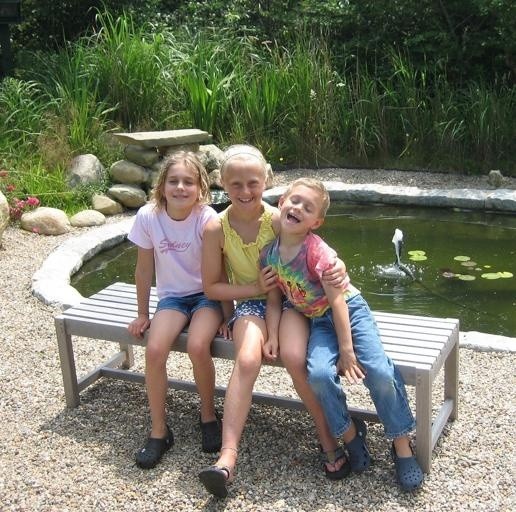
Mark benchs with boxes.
[54,282,459,473]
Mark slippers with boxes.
[198,465,230,498]
[199,411,222,453]
[320,417,369,480]
[391,440,424,491]
[135,424,175,469]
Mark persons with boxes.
[128,150,234,469]
[198,146,351,496]
[255,178,422,492]
